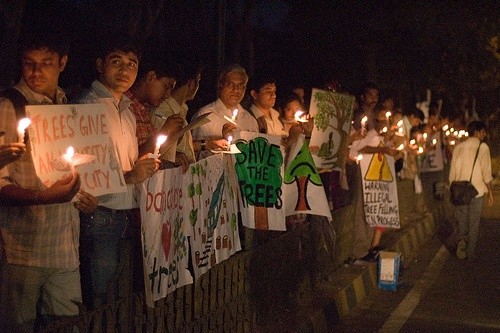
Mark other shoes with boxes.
[455,240,467,261]
[369,245,386,261]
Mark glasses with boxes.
[222,82,245,89]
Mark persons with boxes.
[0,24,99,333]
[449,121,494,259]
[136,56,491,328]
[74,33,162,333]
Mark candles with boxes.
[226,135,232,151]
[231,109,238,123]
[64,146,77,178]
[360,116,368,127]
[378,111,469,156]
[356,154,363,162]
[16,117,32,144]
[153,134,169,157]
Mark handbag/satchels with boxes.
[449,180,479,206]
